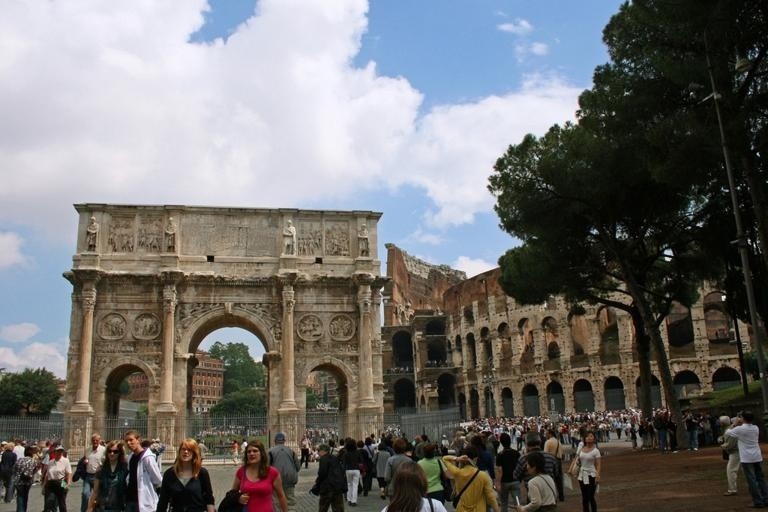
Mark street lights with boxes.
[682,16,766,408]
[483,373,495,419]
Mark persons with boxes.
[165,217,178,251]
[110,218,161,252]
[180,304,194,327]
[298,225,350,256]
[250,304,271,320]
[357,224,370,258]
[282,220,296,255]
[84,216,99,250]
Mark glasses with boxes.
[107,448,120,454]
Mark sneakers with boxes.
[348,500,356,506]
[748,502,765,508]
[358,487,369,497]
[625,437,698,454]
[234,462,240,466]
[561,437,621,448]
[723,490,737,496]
[380,492,386,499]
[300,465,308,469]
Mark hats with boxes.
[54,444,65,453]
[275,431,286,443]
[316,444,330,451]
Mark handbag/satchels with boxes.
[310,482,321,496]
[722,446,730,460]
[96,485,123,510]
[360,463,366,474]
[441,473,460,509]
[568,459,580,478]
